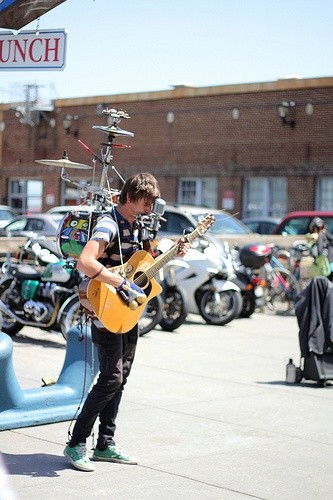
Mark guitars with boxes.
[78,213,216,335]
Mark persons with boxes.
[63,172,191,473]
[283,216,333,315]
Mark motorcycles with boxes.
[0,237,272,337]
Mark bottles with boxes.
[286,359,295,384]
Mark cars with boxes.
[0,205,333,297]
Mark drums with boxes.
[80,186,121,205]
[56,210,90,262]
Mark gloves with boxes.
[117,280,146,308]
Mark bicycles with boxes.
[224,242,304,315]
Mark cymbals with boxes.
[92,125,134,137]
[34,159,93,170]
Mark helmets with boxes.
[310,217,324,233]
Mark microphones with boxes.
[152,198,166,237]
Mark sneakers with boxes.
[93,444,138,465]
[63,441,95,471]
